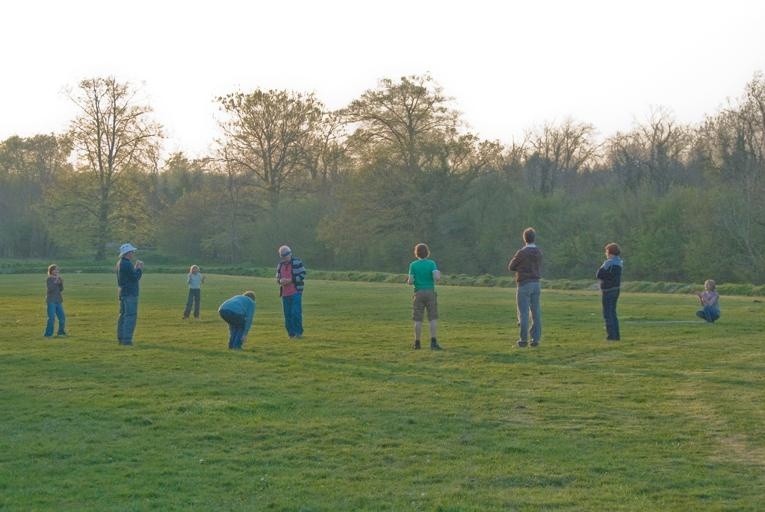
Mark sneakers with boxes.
[411,343,419,349]
[432,343,439,348]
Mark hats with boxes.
[119,244,136,256]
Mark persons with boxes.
[44,264,66,338]
[695,279,720,323]
[183,265,205,321]
[509,227,544,347]
[276,245,306,340]
[596,243,624,341]
[408,243,442,351]
[218,291,256,350]
[115,244,144,347]
